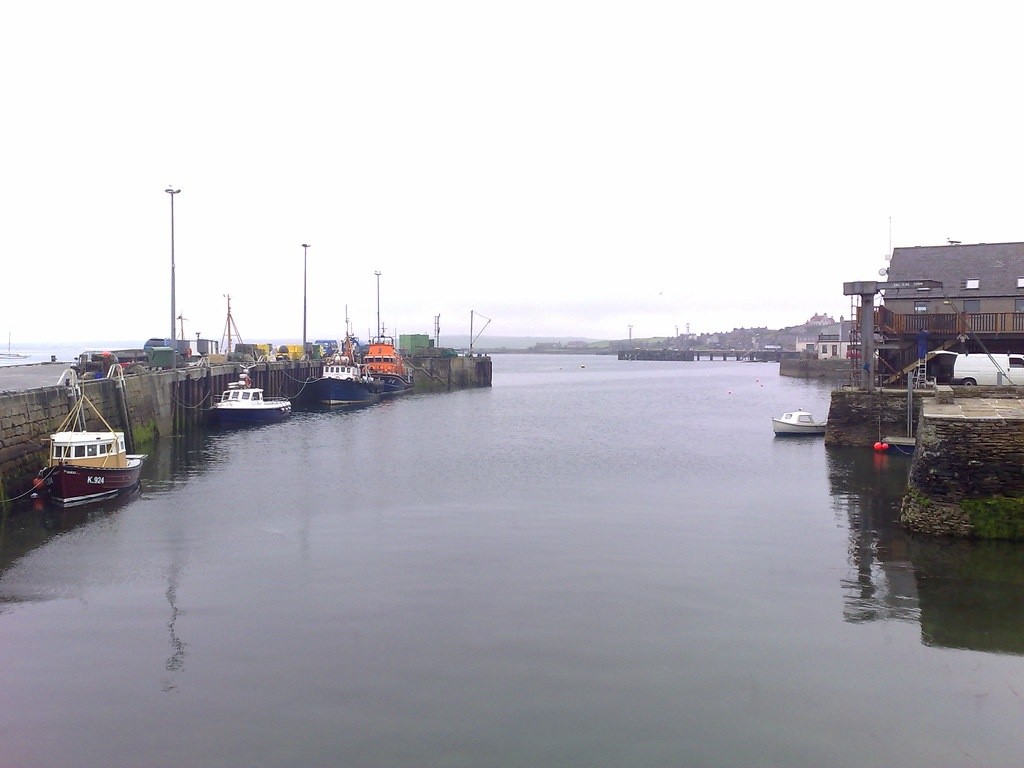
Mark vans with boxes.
[927,350,1024,385]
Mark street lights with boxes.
[374,270,382,342]
[164,188,182,371]
[301,243,311,356]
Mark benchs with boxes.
[934,385,954,404]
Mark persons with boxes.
[914,328,928,359]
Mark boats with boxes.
[310,329,385,406]
[209,364,292,422]
[362,334,414,392]
[771,410,827,435]
[31,393,148,513]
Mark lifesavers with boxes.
[244,377,252,389]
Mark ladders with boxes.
[917,354,927,388]
